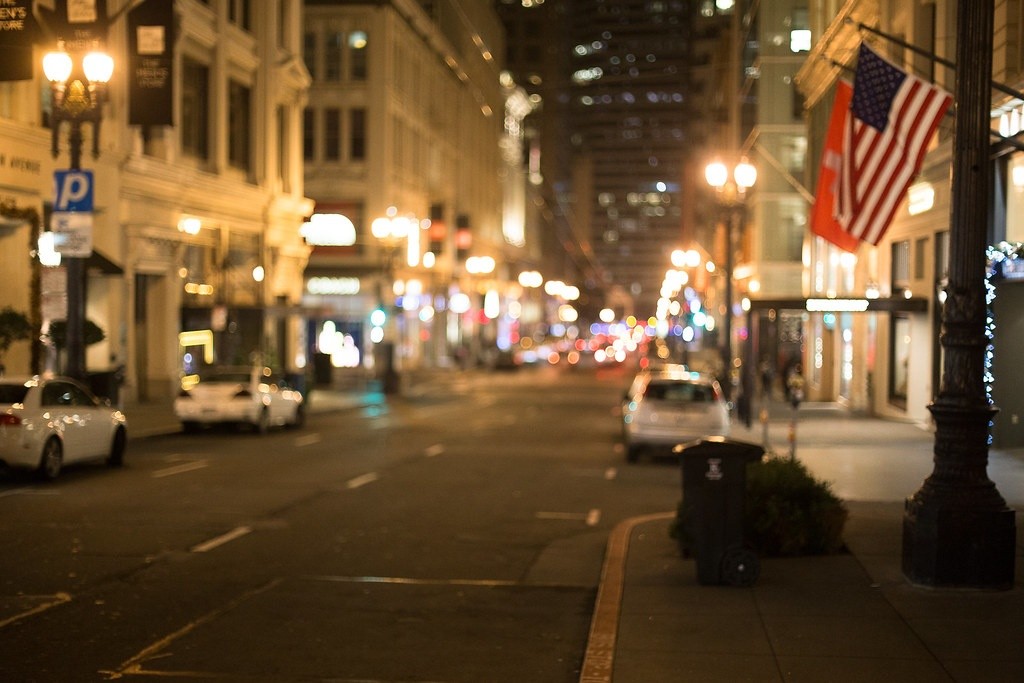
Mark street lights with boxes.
[43,51,114,382]
[705,159,757,405]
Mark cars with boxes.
[0,374,129,484]
[174,365,306,438]
[620,369,731,466]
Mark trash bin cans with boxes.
[89,372,120,407]
[674,436,766,586]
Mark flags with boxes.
[809,76,861,254]
[832,43,955,247]
